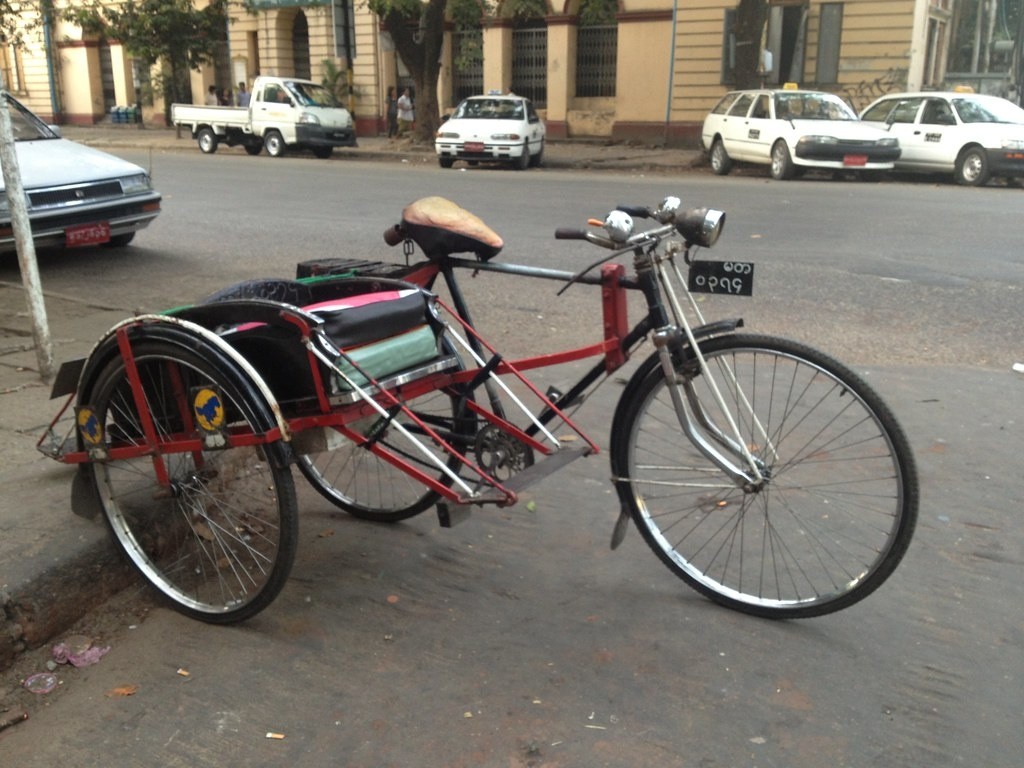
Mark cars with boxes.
[0,90,161,275]
[703,83,902,181]
[435,93,546,169]
[858,86,1024,186]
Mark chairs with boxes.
[776,100,796,119]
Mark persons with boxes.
[202,80,251,108]
[383,85,414,140]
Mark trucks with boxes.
[171,75,357,160]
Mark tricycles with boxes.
[39,196,920,625]
